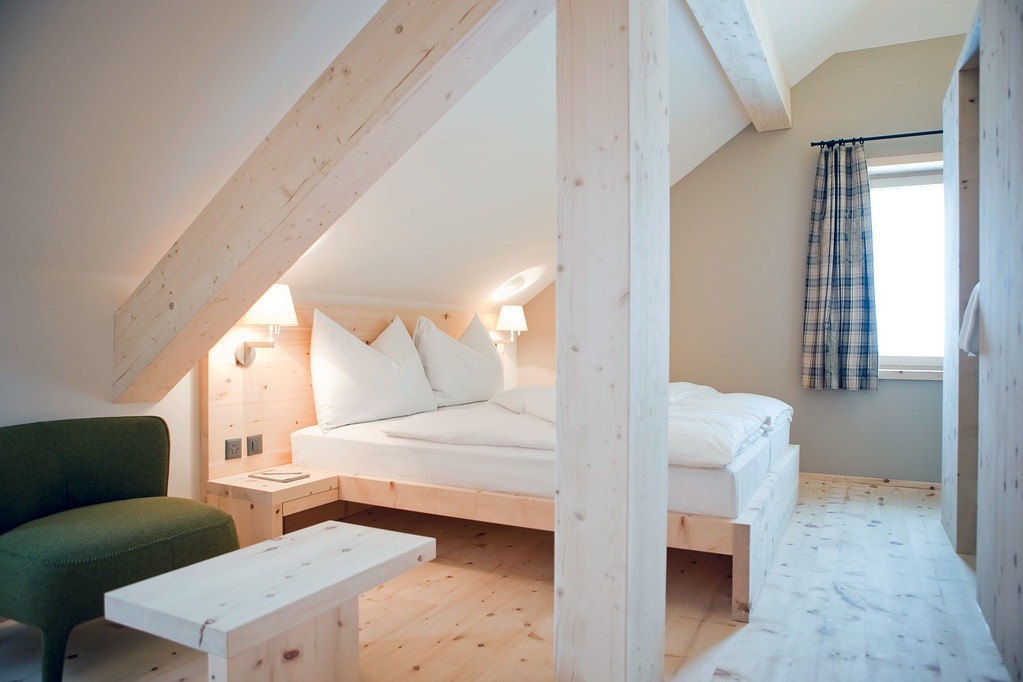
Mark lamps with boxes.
[234,283,297,368]
[493,306,529,347]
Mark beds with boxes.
[194,317,802,622]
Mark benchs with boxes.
[0,417,240,682]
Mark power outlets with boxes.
[225,438,242,460]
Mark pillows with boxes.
[414,315,503,407]
[309,309,437,436]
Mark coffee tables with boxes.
[103,520,435,682]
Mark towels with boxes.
[957,280,981,357]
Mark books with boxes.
[248,468,310,483]
